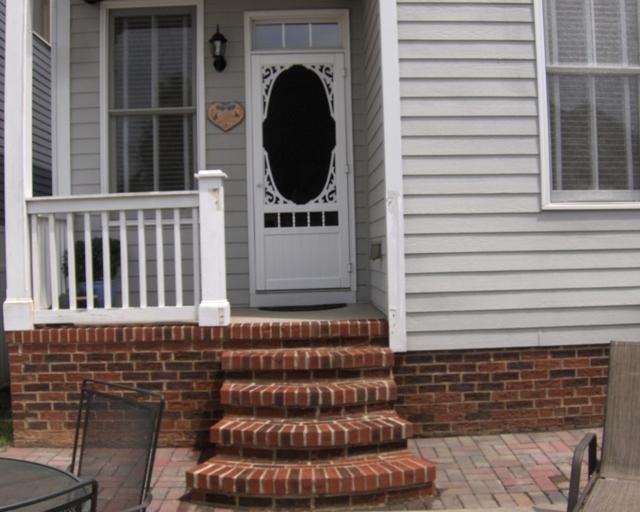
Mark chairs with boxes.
[2,479,98,512]
[566,338,638,511]
[66,378,167,511]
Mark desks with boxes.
[1,454,92,512]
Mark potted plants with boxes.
[62,235,121,307]
